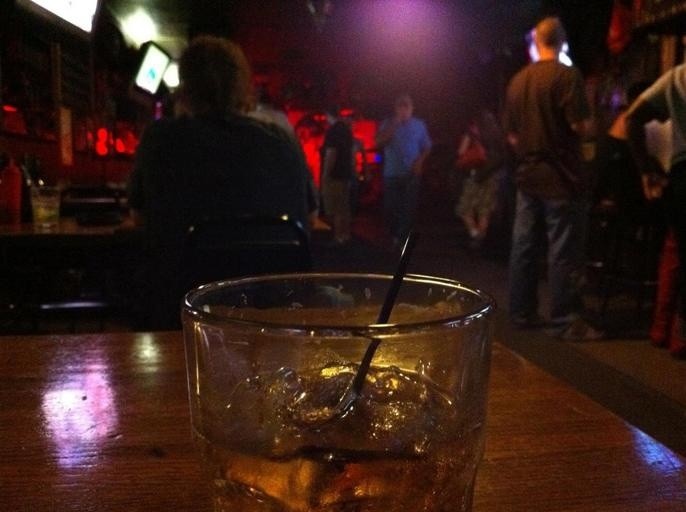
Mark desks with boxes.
[0,211,142,256]
[0,329,686,510]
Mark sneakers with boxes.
[511,311,543,328]
[323,237,354,251]
[555,319,606,342]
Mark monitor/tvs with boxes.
[524,27,574,67]
[15,0,104,42]
[133,41,171,95]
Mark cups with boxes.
[174,272,502,506]
[26,185,61,230]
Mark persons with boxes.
[373,94,436,265]
[128,37,320,332]
[317,104,357,253]
[341,117,370,227]
[451,18,685,366]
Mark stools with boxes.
[1,294,118,333]
